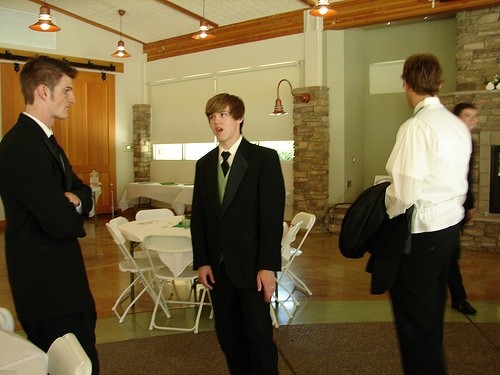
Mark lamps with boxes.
[29,0,61,32]
[484,75,500,91]
[111,10,131,58]
[310,0,338,17]
[192,0,217,40]
[270,79,311,117]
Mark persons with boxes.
[386,53,472,375]
[0,54,100,375]
[451,102,479,315]
[190,93,286,375]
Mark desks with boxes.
[118,182,194,215]
[119,216,288,308]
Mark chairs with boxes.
[268,211,316,329]
[105,208,213,334]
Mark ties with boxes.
[49,135,67,177]
[220,151,231,177]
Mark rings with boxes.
[271,287,274,288]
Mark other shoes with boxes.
[451,301,477,314]
